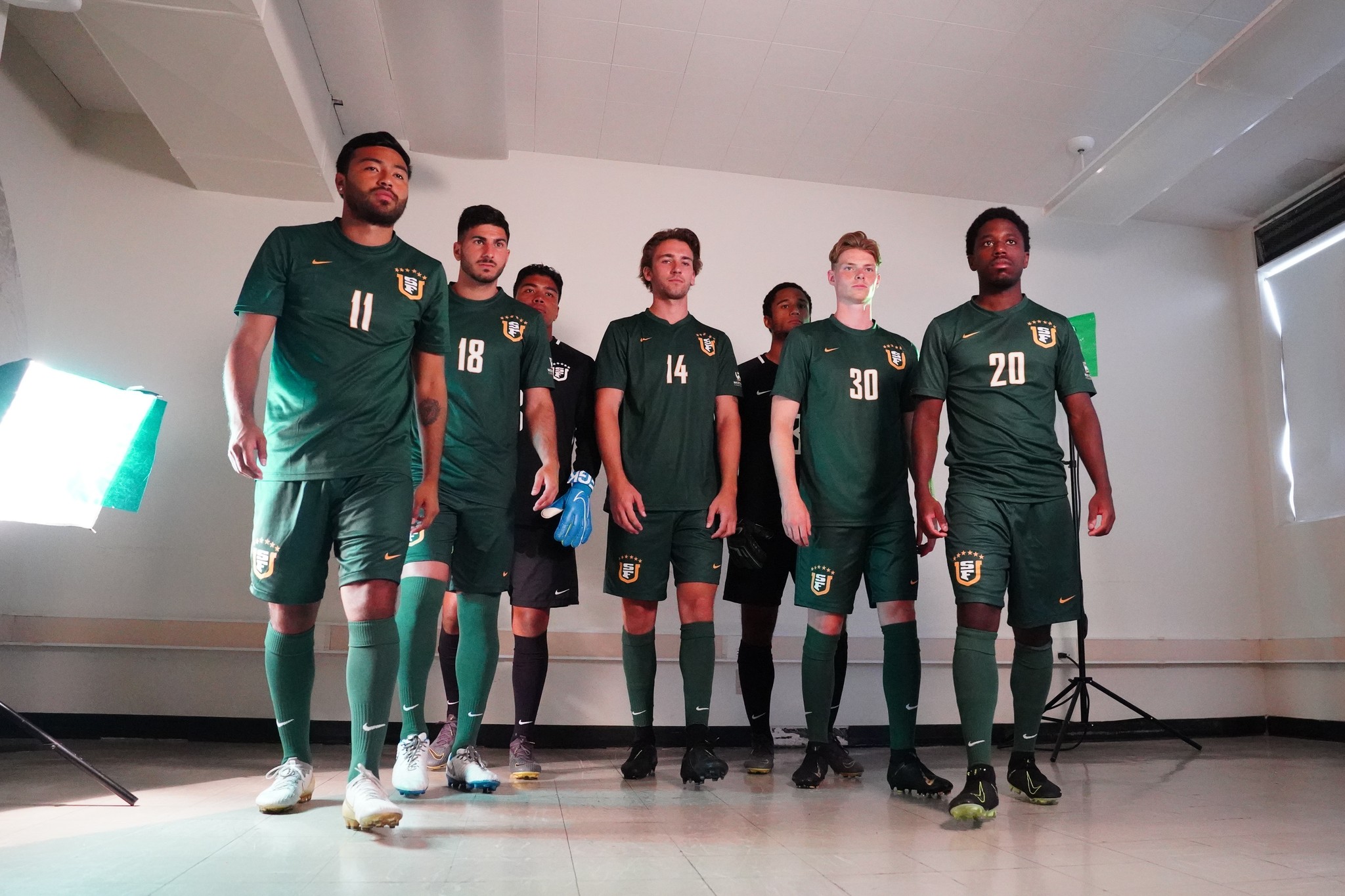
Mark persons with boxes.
[592,228,741,780]
[391,205,560,794]
[425,263,594,780]
[912,206,1117,821]
[722,281,864,778]
[766,230,954,798]
[221,130,450,829]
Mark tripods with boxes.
[1038,396,1203,762]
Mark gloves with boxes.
[541,471,597,551]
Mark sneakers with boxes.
[1005,751,1063,804]
[948,763,999,822]
[620,725,659,778]
[791,739,832,788]
[680,724,729,784]
[743,723,774,773]
[390,733,430,797]
[509,734,541,779]
[888,746,953,799]
[427,714,458,770]
[256,757,315,814]
[828,734,865,777]
[445,745,501,794]
[341,764,404,833]
